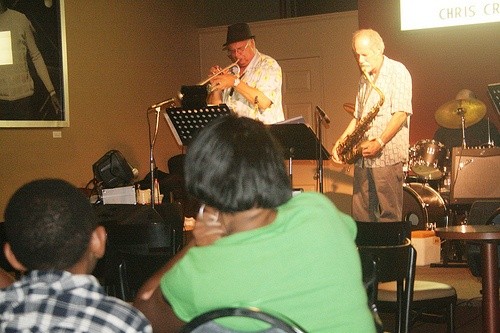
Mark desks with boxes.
[435,223,500,333]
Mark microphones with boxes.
[148,97,176,109]
[315,106,330,124]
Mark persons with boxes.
[434,89,500,152]
[0,179,154,333]
[331,30,412,222]
[0,0,62,121]
[206,23,284,123]
[133,117,376,333]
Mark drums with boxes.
[378,182,449,231]
[406,138,450,193]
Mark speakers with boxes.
[450,147,500,207]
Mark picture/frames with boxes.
[0,0,70,127]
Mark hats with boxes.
[223,22,256,46]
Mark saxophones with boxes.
[330,66,386,166]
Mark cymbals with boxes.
[433,97,488,130]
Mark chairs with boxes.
[180,307,305,333]
[352,221,454,333]
[358,244,416,333]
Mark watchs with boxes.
[51,91,56,97]
[375,136,385,151]
[234,78,240,89]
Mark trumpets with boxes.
[175,57,241,103]
